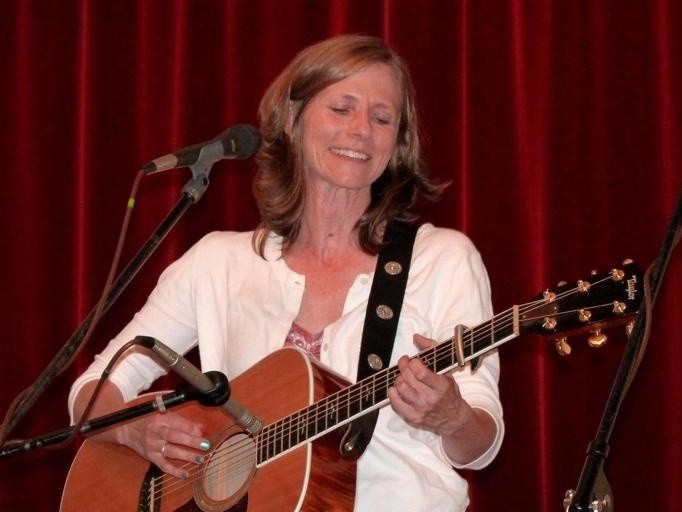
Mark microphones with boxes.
[139,122,261,175]
[150,339,262,436]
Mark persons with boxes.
[65,34,518,512]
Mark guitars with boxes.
[59,259,644,512]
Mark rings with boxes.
[160,443,166,455]
